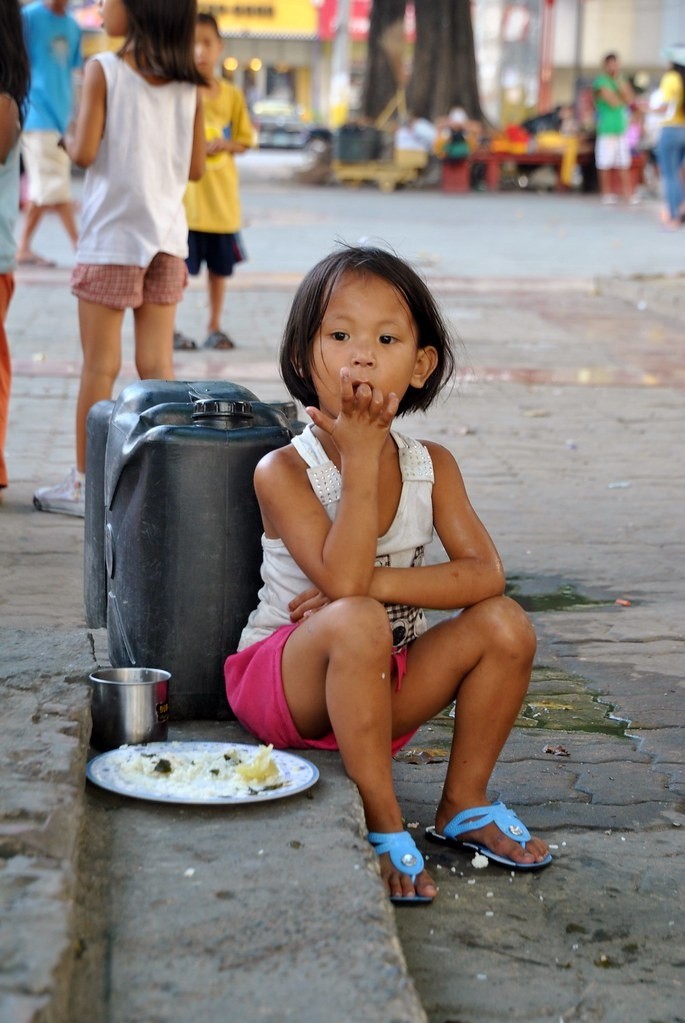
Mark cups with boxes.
[88,668,172,754]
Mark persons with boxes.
[647,42,685,231]
[0,0,32,509]
[33,0,206,520]
[223,247,553,903]
[171,14,255,351]
[593,53,641,208]
[17,0,85,263]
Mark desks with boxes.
[477,150,593,194]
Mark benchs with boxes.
[336,160,416,193]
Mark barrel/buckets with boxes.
[85,378,316,721]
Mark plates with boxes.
[85,741,320,806]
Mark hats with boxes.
[660,43,685,66]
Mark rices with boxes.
[114,742,282,797]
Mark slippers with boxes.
[424,800,552,872]
[366,831,437,905]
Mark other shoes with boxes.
[203,331,234,351]
[34,469,85,518]
[14,251,48,266]
[172,334,197,351]
[659,208,680,230]
[628,195,641,205]
[602,194,616,205]
[678,201,685,216]
